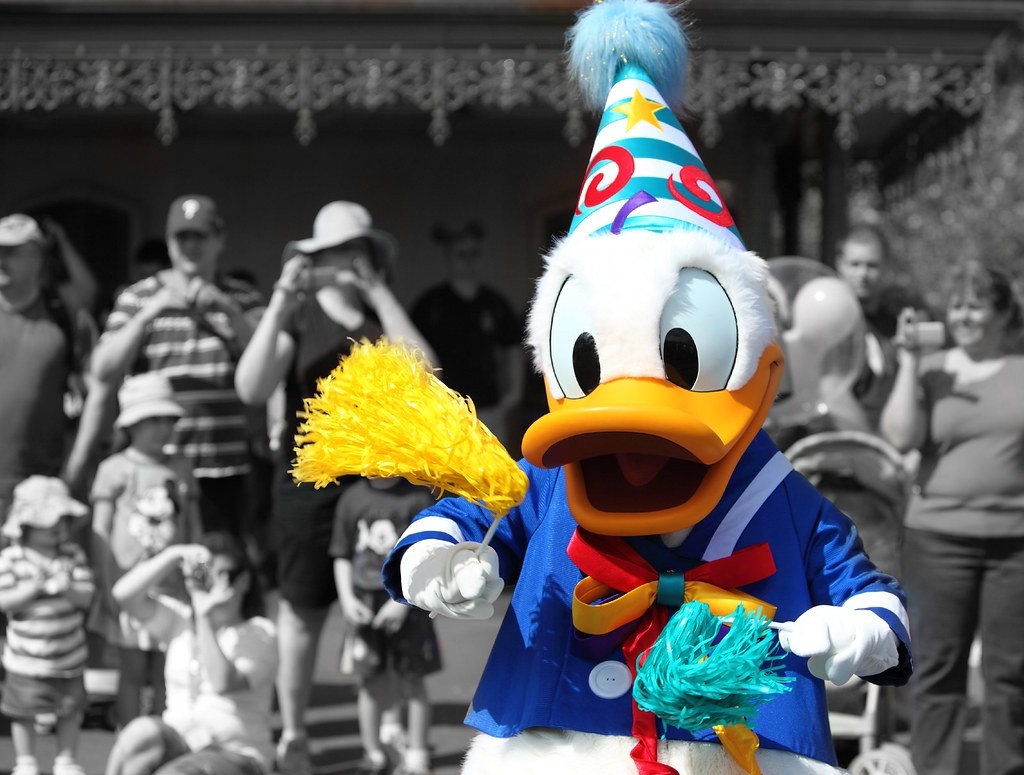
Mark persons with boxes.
[0,194,528,775]
[831,220,1024,775]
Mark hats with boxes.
[168,193,222,238]
[281,200,396,286]
[0,213,60,259]
[113,372,185,431]
[0,475,90,538]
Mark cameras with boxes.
[186,561,212,594]
[904,321,946,352]
[299,266,337,291]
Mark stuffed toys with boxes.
[380,0,913,775]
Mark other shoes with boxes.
[53,763,86,775]
[359,751,389,775]
[11,764,42,775]
[276,726,312,775]
[392,745,431,775]
